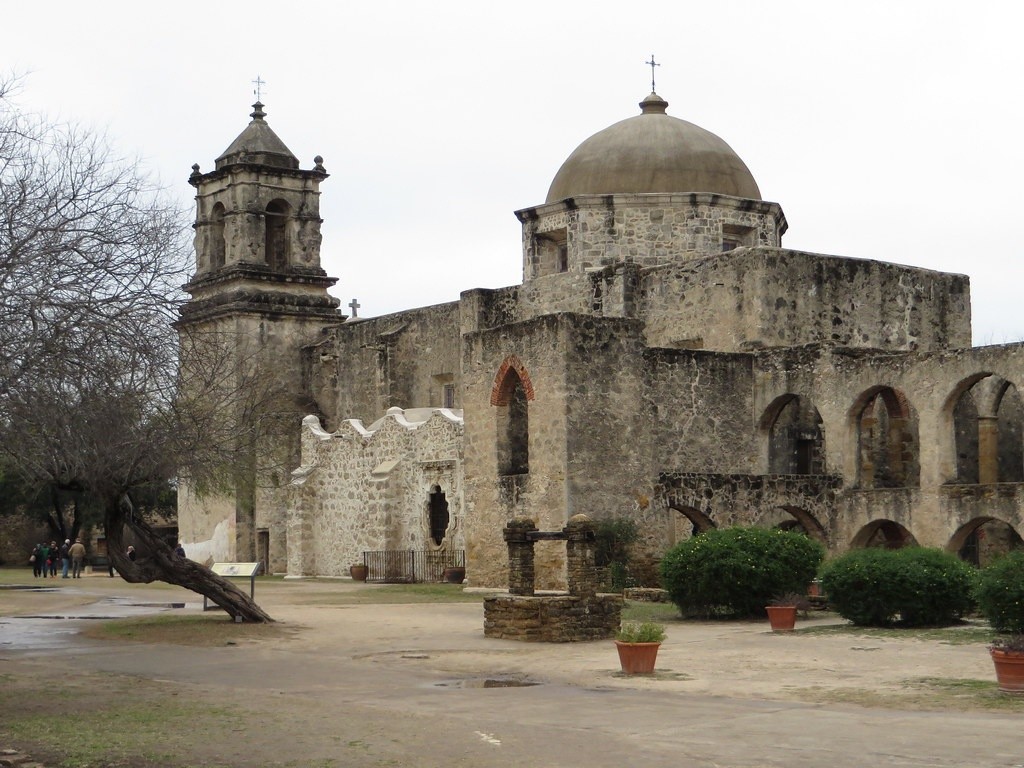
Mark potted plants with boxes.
[351,562,369,581]
[613,622,667,674]
[764,592,812,632]
[445,559,465,584]
[985,631,1024,693]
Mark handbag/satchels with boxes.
[29,555,35,561]
[47,559,52,565]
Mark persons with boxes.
[60,539,71,578]
[126,546,136,560]
[33,542,59,578]
[31,544,44,577]
[175,544,187,558]
[67,537,86,578]
[48,541,60,578]
